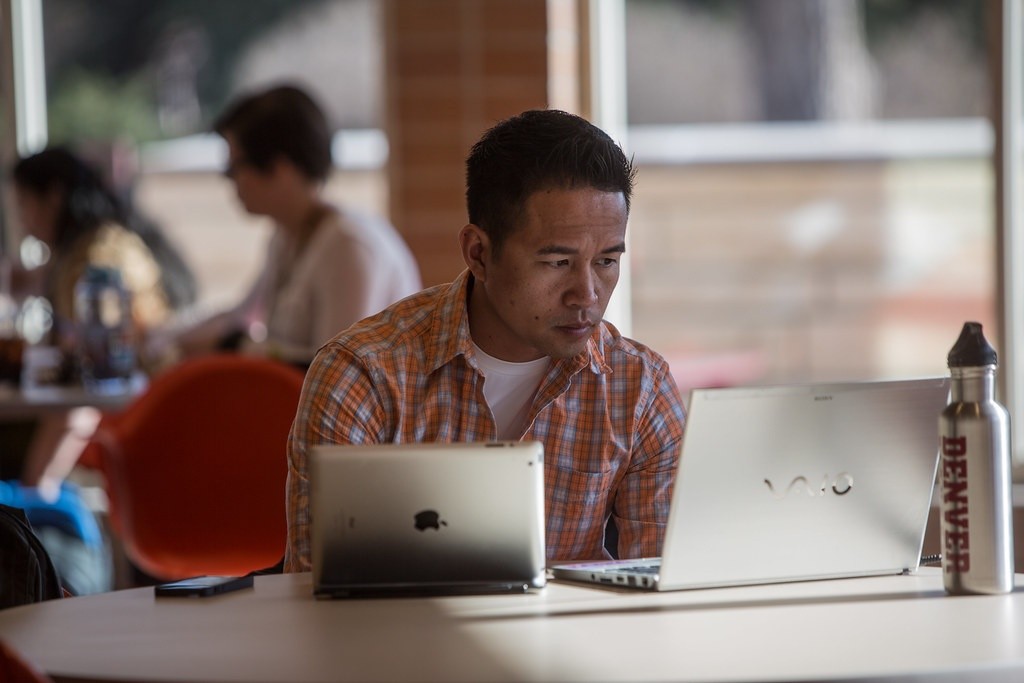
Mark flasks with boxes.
[936,322,1013,595]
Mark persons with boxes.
[2,88,422,589]
[281,110,689,573]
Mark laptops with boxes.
[304,440,550,599]
[554,378,956,594]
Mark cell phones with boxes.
[154,574,255,598]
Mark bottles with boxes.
[72,263,136,392]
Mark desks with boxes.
[0,556,1024,683]
[0,374,149,416]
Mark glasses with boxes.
[225,157,246,178]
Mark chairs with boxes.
[106,355,311,582]
